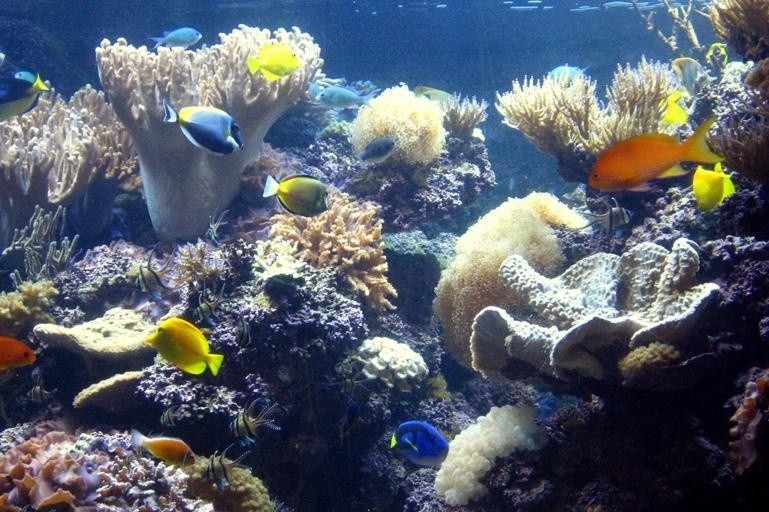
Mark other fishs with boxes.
[133,207,281,494]
